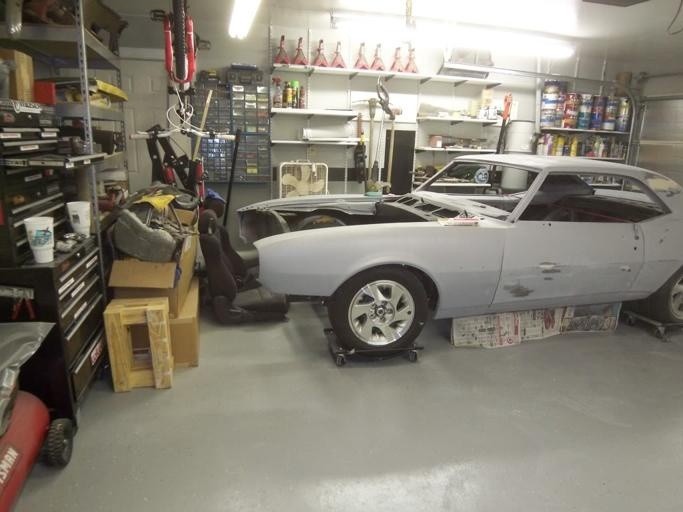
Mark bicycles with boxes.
[128,1,236,141]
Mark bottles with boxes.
[271,35,417,74]
[535,133,577,158]
[282,79,305,109]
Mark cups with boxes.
[66,201,91,241]
[23,215,55,265]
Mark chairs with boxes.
[198,208,292,324]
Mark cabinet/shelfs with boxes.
[270,64,497,187]
[540,126,629,160]
[1,25,132,228]
[0,235,109,426]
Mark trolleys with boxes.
[0,285,73,512]
[409,163,489,184]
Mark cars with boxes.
[235,153,682,358]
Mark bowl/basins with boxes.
[56,240,76,252]
[63,233,84,243]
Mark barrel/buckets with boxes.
[536,92,630,159]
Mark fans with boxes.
[277,161,328,201]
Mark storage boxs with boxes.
[109,233,196,318]
[125,275,199,368]
